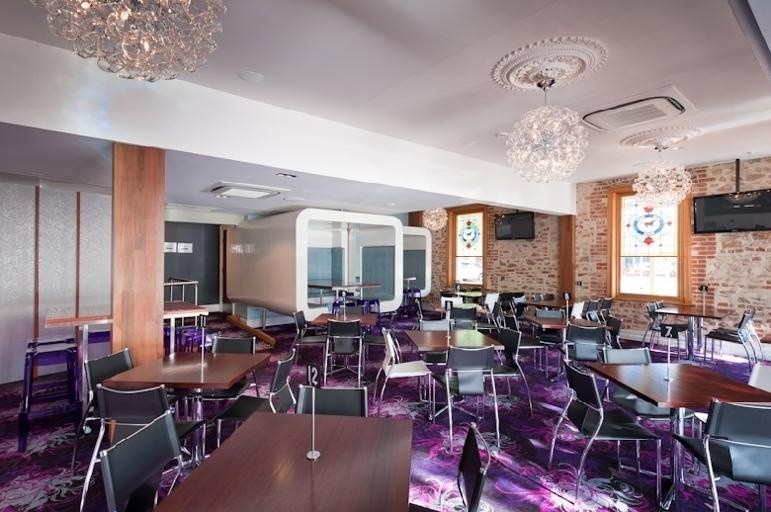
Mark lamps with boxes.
[422,208,448,230]
[619,122,703,207]
[490,35,609,182]
[33,1,227,83]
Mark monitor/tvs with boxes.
[494,212,536,239]
[691,189,771,234]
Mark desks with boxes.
[523,297,577,310]
[404,328,506,359]
[40,300,209,419]
[581,361,771,511]
[403,275,416,292]
[526,319,608,381]
[153,411,412,512]
[312,313,380,333]
[655,303,736,368]
[102,352,270,472]
[307,279,382,306]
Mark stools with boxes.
[184,329,221,360]
[22,335,78,345]
[19,344,81,452]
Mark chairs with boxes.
[291,310,329,371]
[585,301,602,324]
[570,300,586,320]
[451,306,477,333]
[600,299,613,320]
[431,346,502,452]
[217,349,297,447]
[323,318,367,389]
[100,407,187,511]
[672,398,771,512]
[372,328,433,417]
[48,420,107,512]
[746,363,771,395]
[417,318,450,406]
[65,348,133,473]
[548,360,664,503]
[360,313,402,375]
[535,308,566,379]
[94,384,206,459]
[411,422,492,512]
[563,324,604,365]
[596,310,622,345]
[504,313,549,381]
[339,305,366,315]
[736,319,771,374]
[193,335,259,400]
[655,301,689,329]
[467,327,535,423]
[599,347,684,480]
[296,387,368,419]
[641,303,688,363]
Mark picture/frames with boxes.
[446,203,489,289]
[607,187,694,306]
